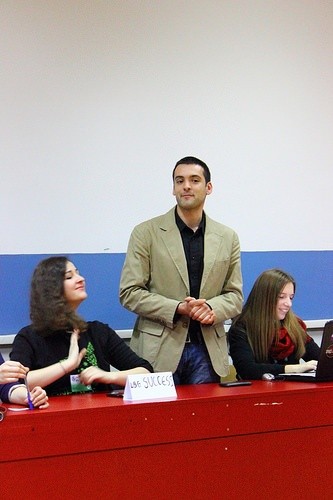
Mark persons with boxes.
[226,269,321,381]
[8,257,154,397]
[0,350,50,409]
[119,156,245,385]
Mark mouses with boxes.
[261,373,275,381]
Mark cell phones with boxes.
[219,381,251,386]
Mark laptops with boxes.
[276,321,333,381]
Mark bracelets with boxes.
[58,361,67,375]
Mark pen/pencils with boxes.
[25,377,34,410]
[106,394,122,398]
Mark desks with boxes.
[0,378,333,500]
